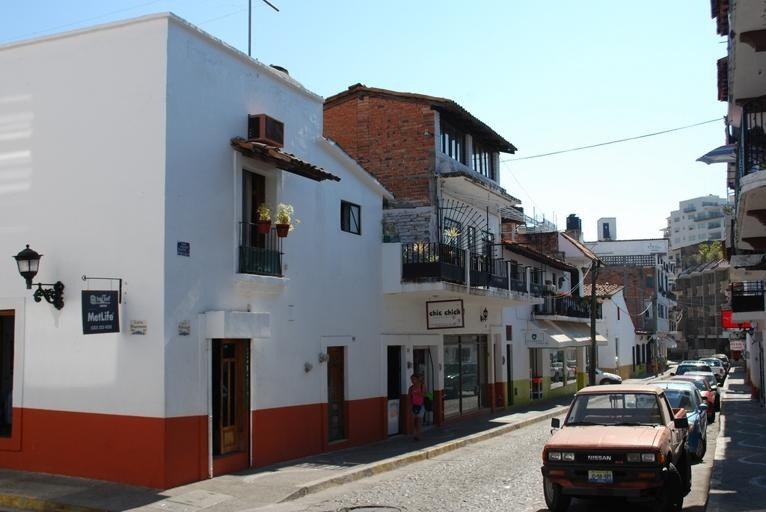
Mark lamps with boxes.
[480,307,488,322]
[11,243,66,311]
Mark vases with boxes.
[256,221,289,238]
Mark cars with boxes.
[444,363,477,396]
[541,351,732,511]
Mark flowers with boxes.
[256,202,300,232]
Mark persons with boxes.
[406,373,426,442]
[658,354,665,375]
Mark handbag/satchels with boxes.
[412,404,421,414]
